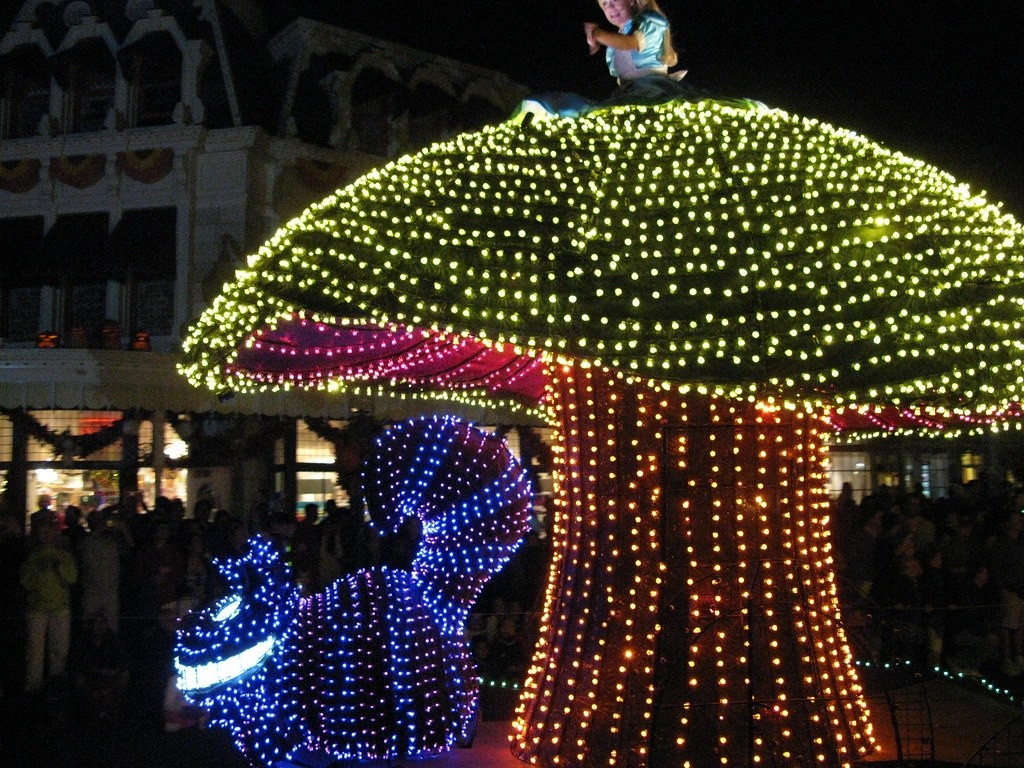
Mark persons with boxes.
[468,529,538,680]
[836,483,1024,672]
[0,491,421,689]
[585,0,677,106]
